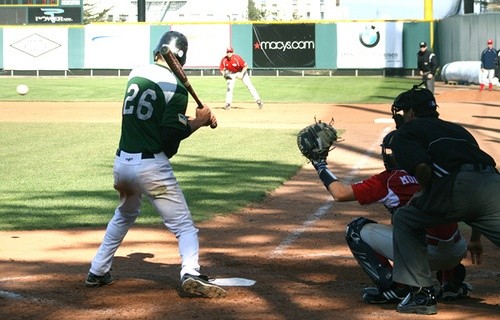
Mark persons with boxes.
[86,31,227,298]
[312,130,469,303]
[220,48,264,110]
[417,42,435,95]
[479,39,497,92]
[392,90,500,315]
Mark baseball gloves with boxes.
[297,116,345,168]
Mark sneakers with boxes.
[181,273,226,298]
[85,268,114,285]
[396,288,437,315]
[257,100,263,109]
[362,286,410,303]
[224,103,230,110]
[442,282,473,298]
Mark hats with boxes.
[488,39,493,43]
[419,42,426,46]
[227,48,233,51]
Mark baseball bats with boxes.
[161,46,217,129]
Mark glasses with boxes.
[227,51,233,53]
[488,43,492,45]
[420,46,426,48]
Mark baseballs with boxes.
[16,84,29,95]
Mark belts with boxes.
[114,149,155,159]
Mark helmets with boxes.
[391,84,439,129]
[380,130,398,170]
[153,31,188,66]
